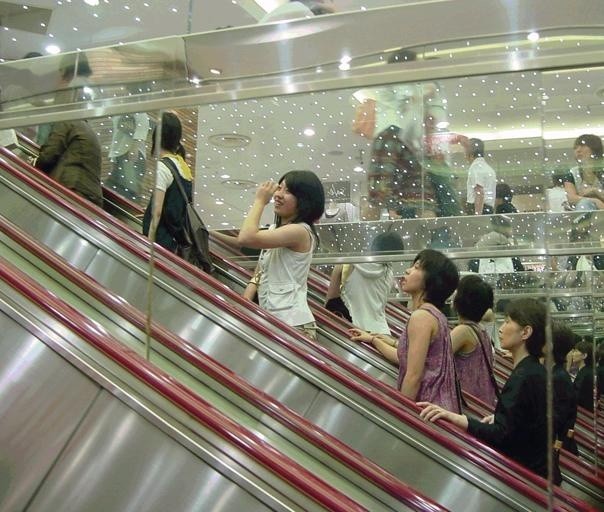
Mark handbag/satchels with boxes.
[351,99,377,138]
[324,296,352,324]
[530,439,563,487]
[171,199,218,277]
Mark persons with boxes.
[325,53,604,273]
[27,107,108,208]
[256,1,333,25]
[50,64,92,106]
[328,232,404,337]
[142,112,218,279]
[541,320,579,454]
[415,297,561,492]
[238,165,324,342]
[480,307,494,338]
[348,249,461,428]
[568,333,604,414]
[374,273,500,414]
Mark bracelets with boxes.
[392,339,397,347]
[246,276,260,284]
[369,336,375,344]
